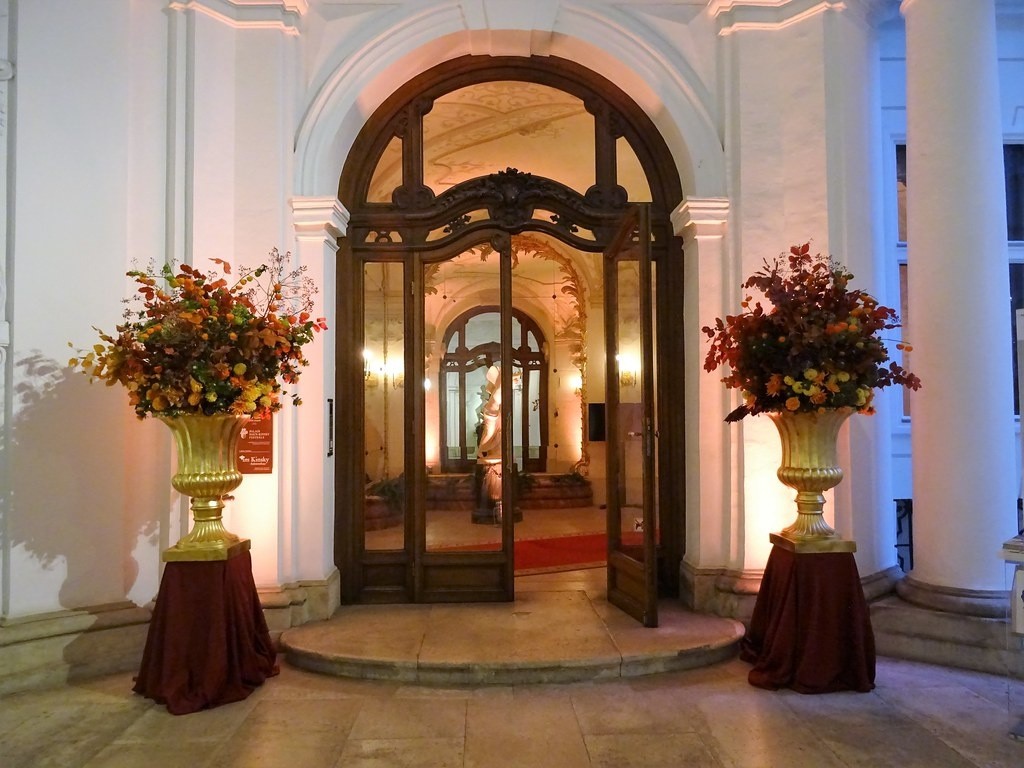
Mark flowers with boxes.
[61,242,326,419]
[696,240,916,425]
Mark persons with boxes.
[472,422,489,505]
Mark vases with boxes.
[150,410,255,562]
[765,405,860,553]
